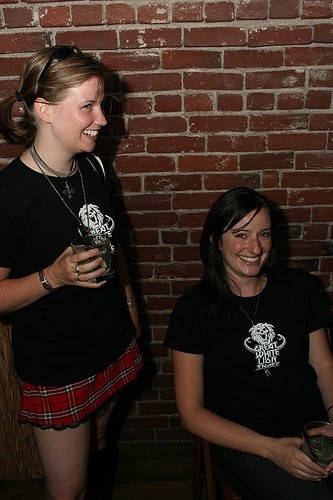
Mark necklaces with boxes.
[31,144,76,200]
[28,147,90,235]
[236,277,262,325]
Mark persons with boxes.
[0,46,147,500]
[162,188,333,500]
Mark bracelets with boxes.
[326,405,333,414]
[38,269,55,292]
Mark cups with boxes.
[70,234,116,283]
[301,420,333,470]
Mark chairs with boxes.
[192,435,241,500]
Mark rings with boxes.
[77,272,82,281]
[75,265,80,272]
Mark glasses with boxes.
[33,44,83,98]
